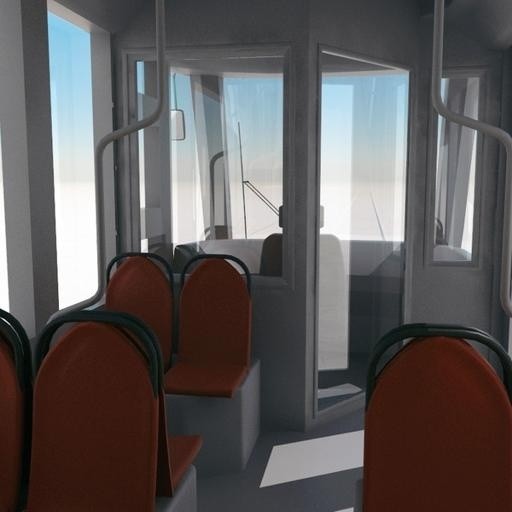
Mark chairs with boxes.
[259,204,345,279]
[359,322,512,512]
